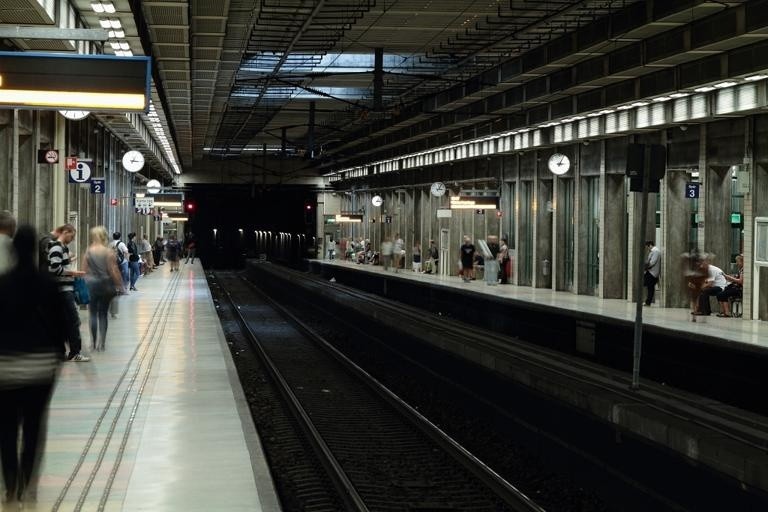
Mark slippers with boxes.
[716,312,731,316]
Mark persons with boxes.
[381,233,404,273]
[0,208,88,510]
[327,236,379,266]
[411,240,440,274]
[82,225,129,351]
[678,242,743,319]
[126,232,141,291]
[643,241,660,308]
[458,236,510,286]
[140,232,196,272]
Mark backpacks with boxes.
[114,241,124,264]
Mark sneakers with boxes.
[643,302,649,306]
[692,311,710,315]
[68,353,90,362]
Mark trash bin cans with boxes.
[484,260,498,284]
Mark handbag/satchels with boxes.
[73,278,90,304]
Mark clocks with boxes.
[146,179,162,194]
[57,109,90,122]
[120,149,146,174]
[371,195,384,207]
[547,153,571,176]
[430,180,447,198]
